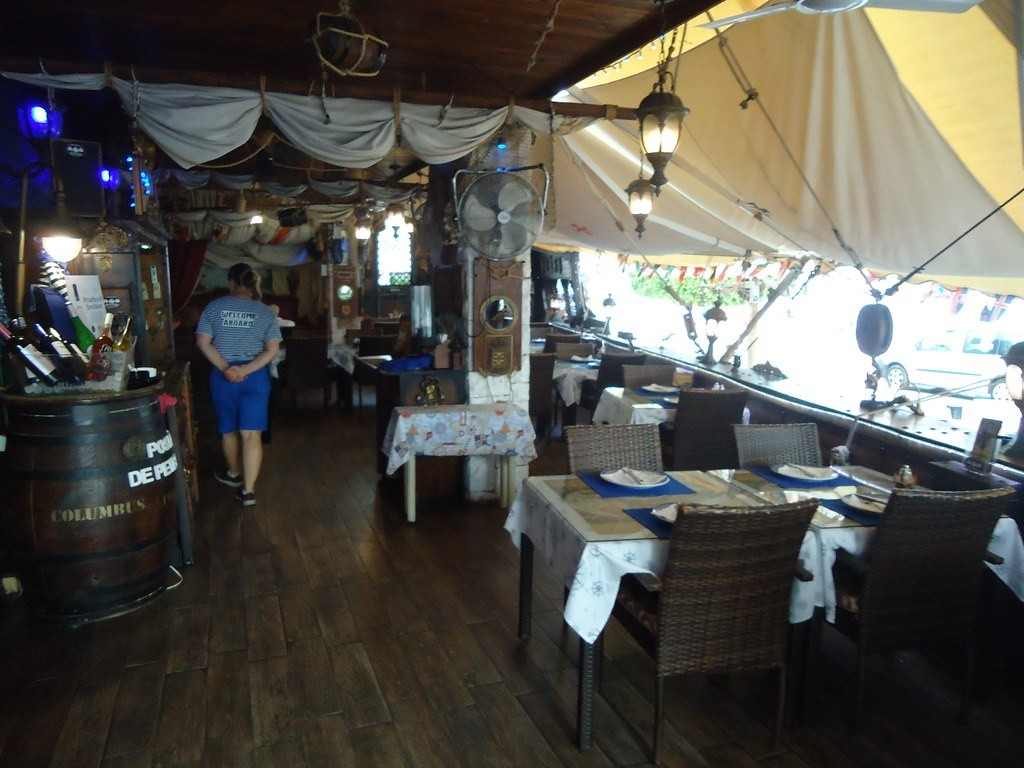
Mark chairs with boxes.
[328,311,1017,767]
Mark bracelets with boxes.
[226,366,230,370]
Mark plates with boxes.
[599,463,670,489]
[842,495,888,514]
[642,384,680,393]
[588,362,600,366]
[652,501,677,523]
[770,462,840,482]
[663,396,679,403]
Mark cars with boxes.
[885,322,1015,402]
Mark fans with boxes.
[450,161,552,259]
[692,0,984,28]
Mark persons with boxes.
[196,263,283,506]
[263,294,298,390]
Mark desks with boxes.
[549,354,602,437]
[382,400,536,526]
[517,462,934,748]
[593,384,751,469]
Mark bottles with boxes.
[898,464,913,488]
[0,301,135,396]
[713,382,725,391]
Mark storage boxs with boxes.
[8,337,139,394]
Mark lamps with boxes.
[601,294,617,332]
[698,296,727,365]
[354,187,373,239]
[643,28,689,198]
[38,183,87,265]
[993,342,1024,458]
[623,169,655,237]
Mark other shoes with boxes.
[213,468,243,486]
[234,490,256,505]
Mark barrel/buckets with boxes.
[0,394,178,622]
